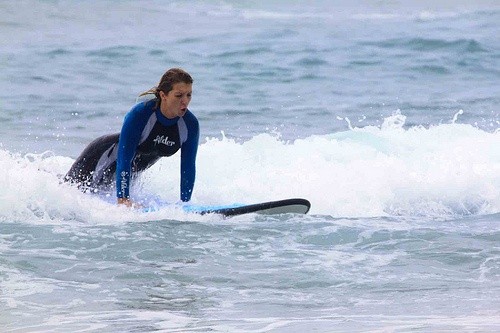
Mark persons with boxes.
[60,69,199,210]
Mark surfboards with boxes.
[136,198,310,218]
